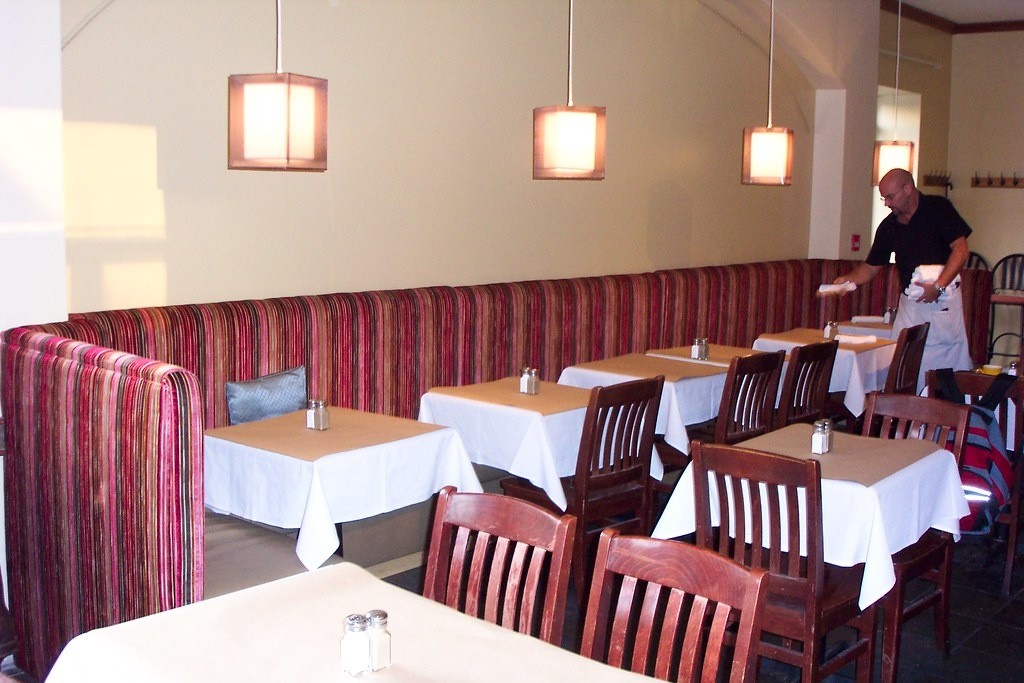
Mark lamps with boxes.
[871,0,915,185]
[228,0,328,172]
[532,1,605,182]
[744,0,795,187]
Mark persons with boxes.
[834,169,974,394]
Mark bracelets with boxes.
[935,284,944,294]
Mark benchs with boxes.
[0,257,993,682]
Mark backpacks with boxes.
[923,367,1019,535]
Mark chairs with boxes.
[422,324,1024,683]
[988,253,1023,358]
[963,251,989,270]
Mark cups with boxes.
[976,365,1002,375]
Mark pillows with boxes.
[226,364,308,424]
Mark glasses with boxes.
[880,183,907,201]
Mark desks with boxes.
[827,322,893,338]
[644,344,813,409]
[650,425,970,607]
[199,407,485,571]
[40,564,673,681]
[748,328,896,419]
[416,373,665,511]
[556,347,765,483]
[912,366,1018,454]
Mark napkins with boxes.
[819,281,861,292]
[850,315,883,322]
[904,264,961,301]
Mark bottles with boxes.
[883,307,897,325]
[812,419,834,454]
[306,399,330,431]
[340,610,392,678]
[824,321,839,340]
[519,367,539,395]
[1008,361,1021,376]
[692,338,710,360]
